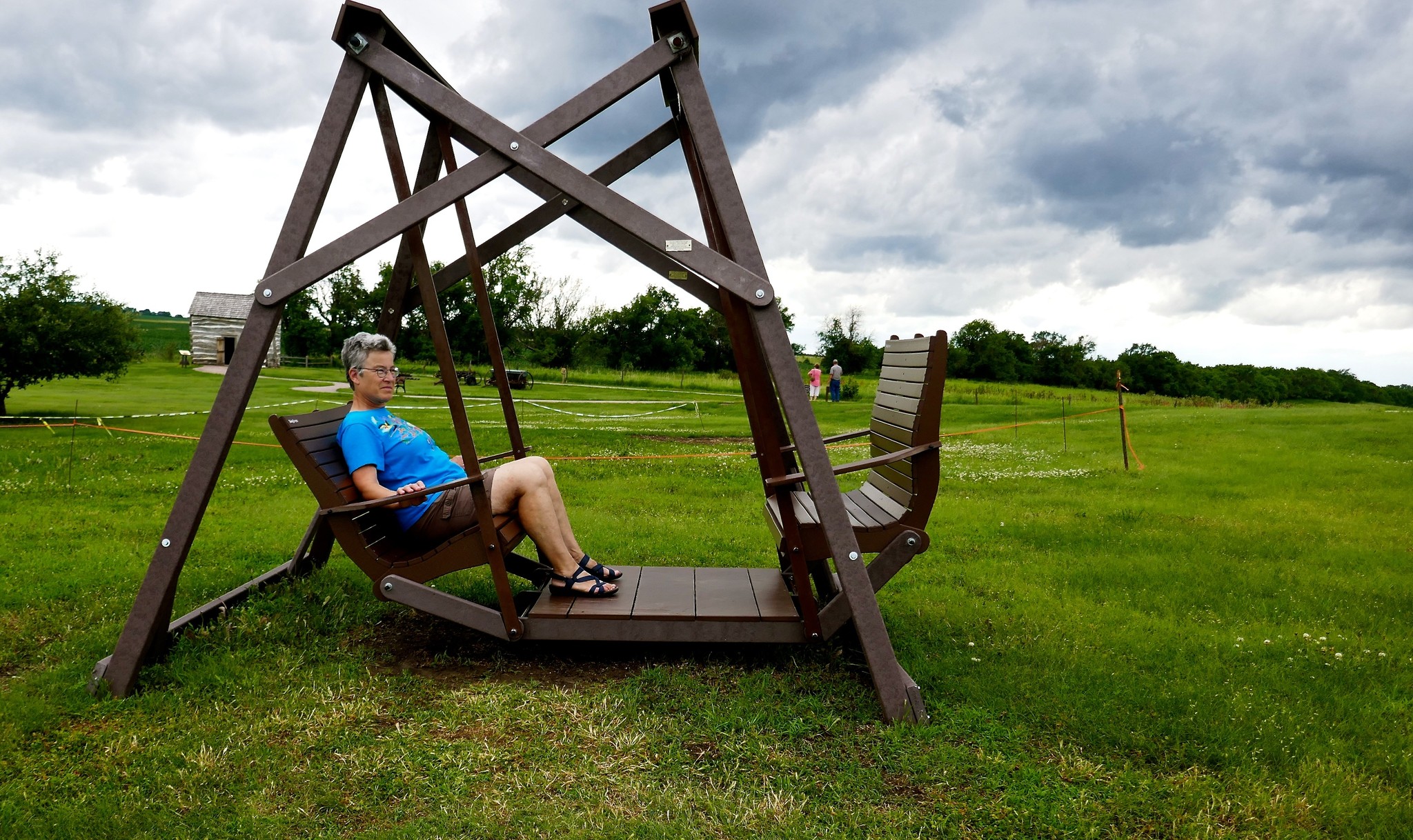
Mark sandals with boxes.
[548,567,619,597]
[567,552,623,582]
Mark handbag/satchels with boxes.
[810,377,815,382]
[833,360,837,363]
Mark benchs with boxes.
[269,403,526,585]
[395,377,407,393]
[762,330,948,565]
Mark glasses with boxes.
[353,366,400,378]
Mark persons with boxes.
[807,364,822,401]
[337,332,622,598]
[827,359,842,402]
